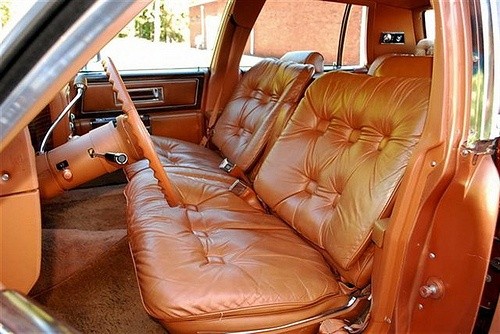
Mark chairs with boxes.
[124,56,435,333]
[123,51,324,190]
